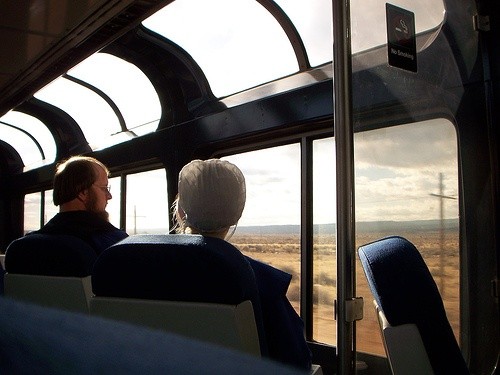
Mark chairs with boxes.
[357,235,471,375]
[4,233,97,315]
[88,234,261,359]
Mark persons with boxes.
[26,155,128,254]
[169,159,312,372]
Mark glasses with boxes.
[92,183,111,192]
